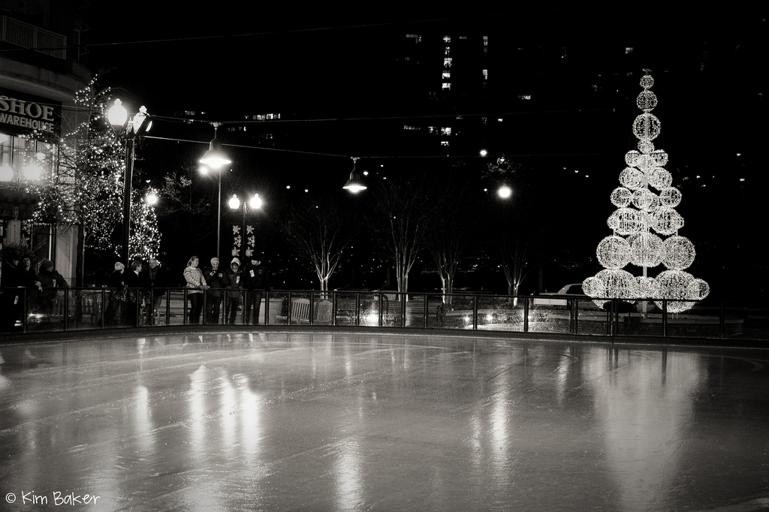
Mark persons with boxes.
[37,258,66,327]
[140,333,272,395]
[98,260,126,326]
[11,255,43,327]
[182,254,210,324]
[202,255,224,324]
[221,256,244,325]
[125,260,145,323]
[241,252,266,325]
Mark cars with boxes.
[528,282,655,313]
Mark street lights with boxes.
[106,95,154,291]
[225,191,269,270]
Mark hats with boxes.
[230,257,240,265]
[114,262,125,271]
